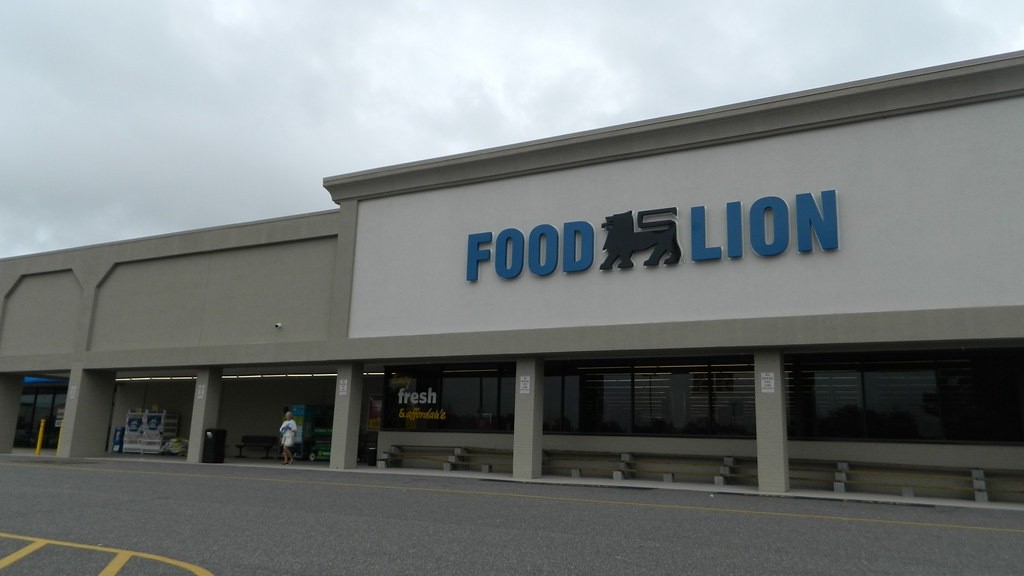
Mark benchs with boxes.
[377,445,1024,503]
[235,435,279,459]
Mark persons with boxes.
[280,412,300,464]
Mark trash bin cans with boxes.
[202,428,227,464]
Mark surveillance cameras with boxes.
[275,323,281,328]
